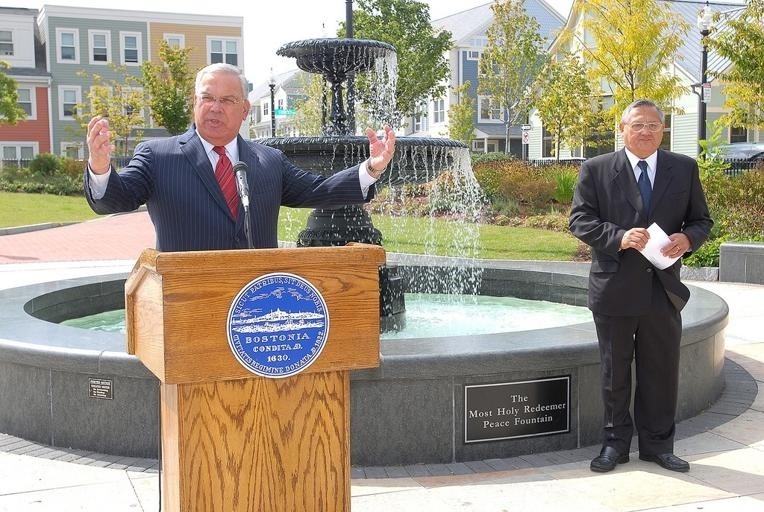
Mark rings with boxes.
[676,245,681,251]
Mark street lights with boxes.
[267,66,279,137]
[320,23,331,136]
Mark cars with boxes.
[708,142,764,170]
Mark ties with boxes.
[213,145,239,222]
[636,160,653,219]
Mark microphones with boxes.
[232,161,250,208]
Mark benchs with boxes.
[719,241,764,285]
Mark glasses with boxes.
[196,92,245,105]
[624,122,663,133]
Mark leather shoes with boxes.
[590,444,629,473]
[639,450,690,472]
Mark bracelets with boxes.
[367,157,387,175]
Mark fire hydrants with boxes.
[697,0,711,161]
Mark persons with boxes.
[83,62,397,253]
[568,98,715,473]
